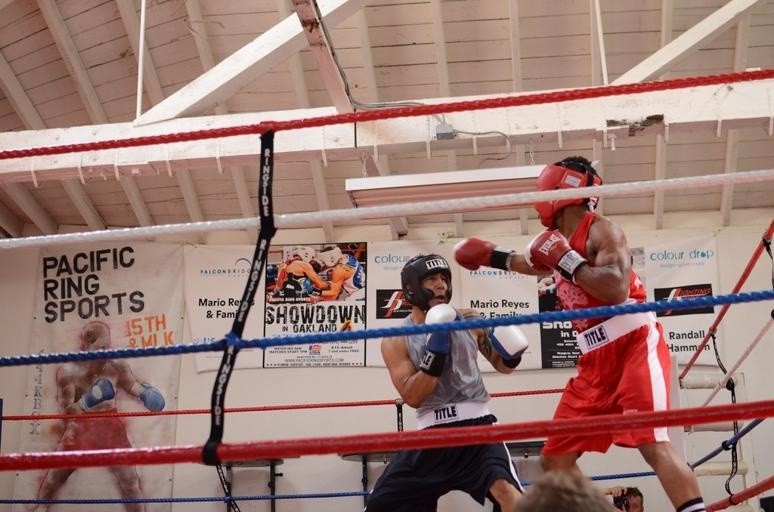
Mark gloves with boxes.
[309,286,323,297]
[487,325,529,369]
[524,230,588,286]
[79,379,116,412]
[453,237,516,272]
[129,378,165,412]
[418,303,461,378]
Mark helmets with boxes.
[399,252,453,310]
[317,246,343,267]
[293,247,315,264]
[532,163,604,228]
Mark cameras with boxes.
[613,490,630,512]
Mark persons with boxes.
[362,251,528,512]
[454,155,708,512]
[512,468,616,512]
[537,274,557,299]
[601,485,645,512]
[268,245,366,302]
[33,322,165,512]
